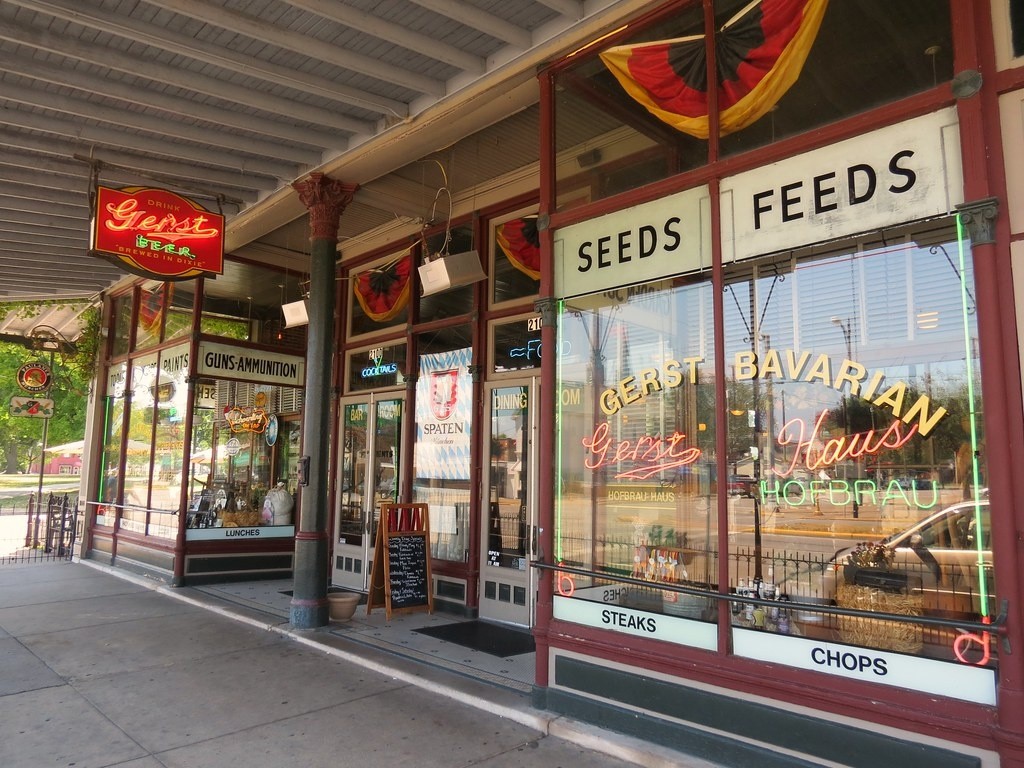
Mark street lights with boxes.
[830,317,860,520]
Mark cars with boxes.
[894,473,933,492]
[727,474,755,498]
[762,475,803,497]
[823,502,991,619]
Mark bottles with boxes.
[736,577,792,635]
[200,499,222,528]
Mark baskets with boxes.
[219,496,259,527]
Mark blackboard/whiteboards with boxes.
[368,503,433,613]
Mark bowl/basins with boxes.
[327,592,361,622]
